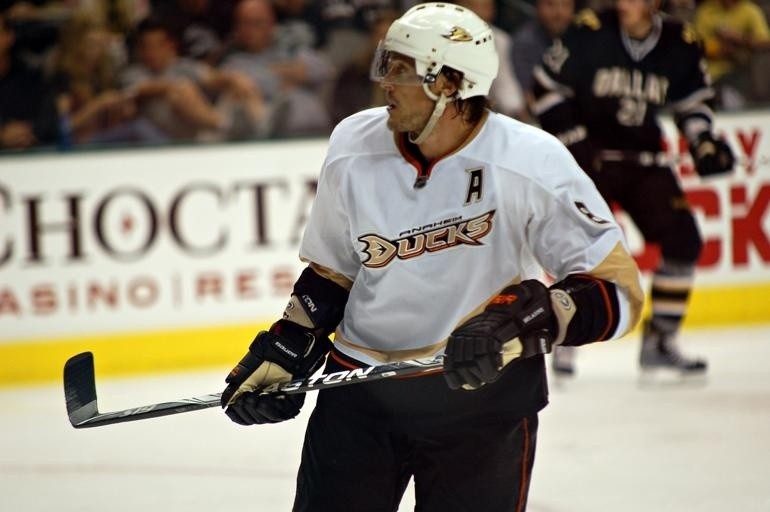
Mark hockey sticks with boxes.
[63,344,447,429]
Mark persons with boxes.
[219,2,647,511]
[1,2,770,150]
[526,0,736,376]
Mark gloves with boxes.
[676,113,732,175]
[538,101,603,165]
[443,279,558,391]
[221,319,334,426]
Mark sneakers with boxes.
[640,330,707,373]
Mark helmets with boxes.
[369,2,499,102]
